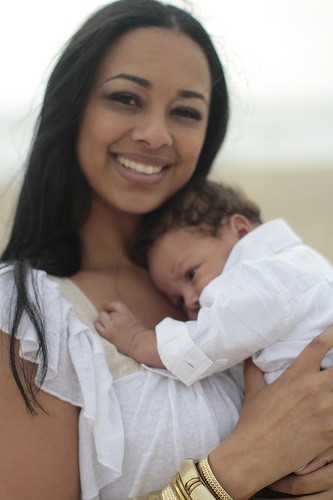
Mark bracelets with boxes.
[146,454,235,500]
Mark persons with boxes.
[93,179,333,385]
[0,1,333,500]
